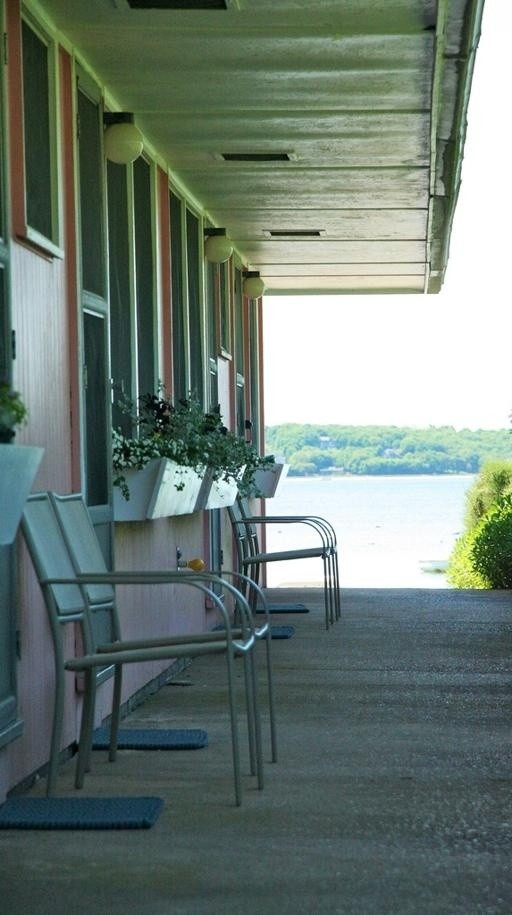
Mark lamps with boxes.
[103,110,145,167]
[242,271,266,300]
[204,226,234,265]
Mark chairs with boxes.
[224,492,343,629]
[51,493,277,775]
[22,494,264,807]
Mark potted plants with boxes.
[1,384,49,552]
[250,453,284,499]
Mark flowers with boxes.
[107,376,278,497]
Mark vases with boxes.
[112,457,247,523]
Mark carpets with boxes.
[211,624,295,641]
[1,796,165,831]
